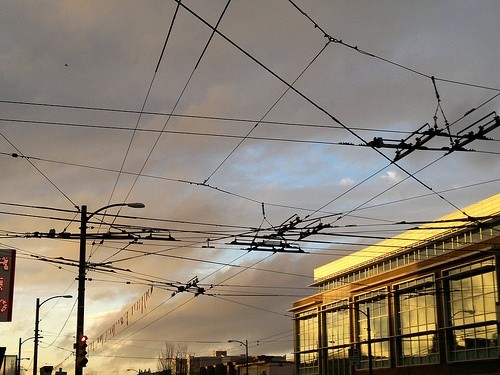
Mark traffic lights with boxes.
[78,336,87,368]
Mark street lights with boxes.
[18,358,30,374]
[33,294,73,375]
[166,357,182,375]
[127,368,140,375]
[228,340,249,375]
[18,336,43,375]
[339,306,373,374]
[75,202,145,375]
